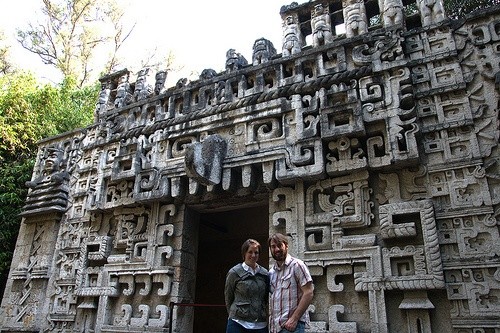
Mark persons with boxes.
[269,233,314,333]
[225,239,269,333]
